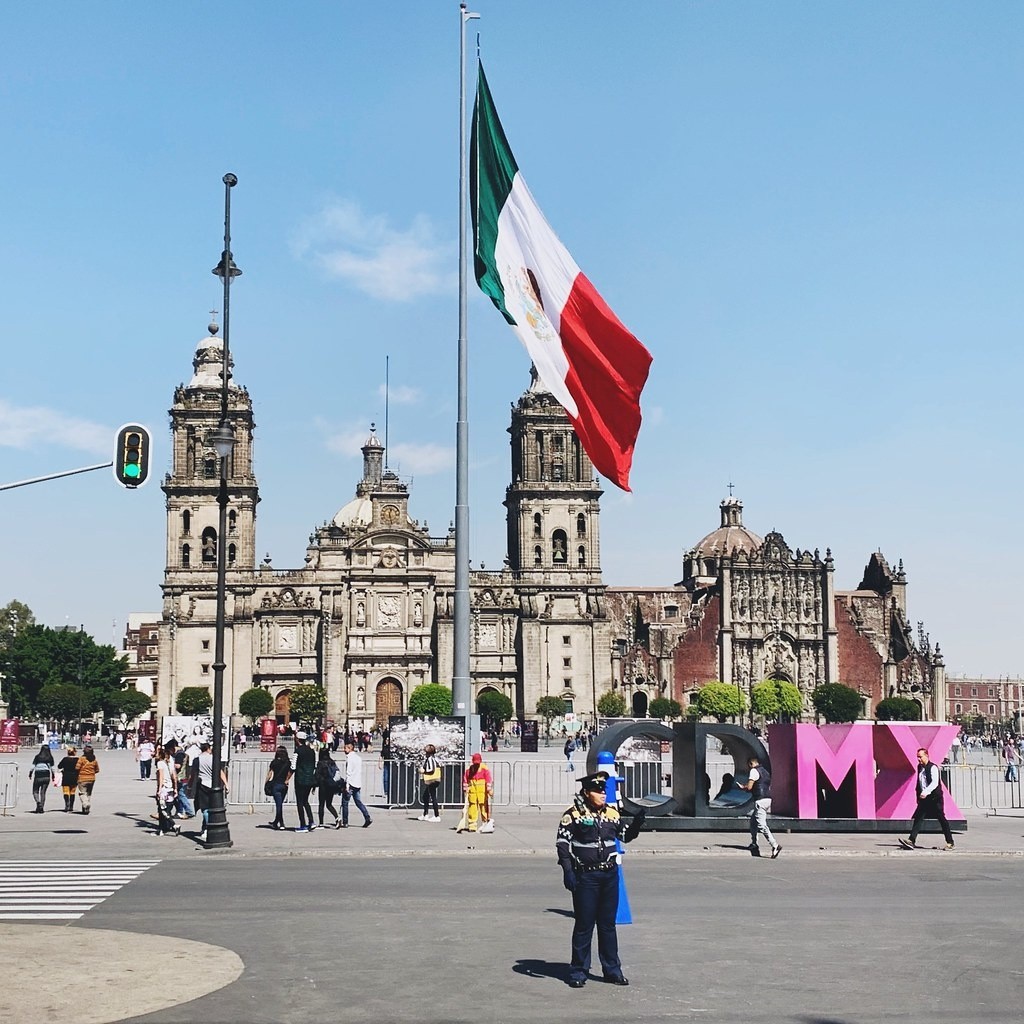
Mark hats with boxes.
[295,732,307,740]
[575,771,609,789]
[472,753,482,764]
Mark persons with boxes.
[463,753,492,831]
[75,746,99,814]
[62,725,371,752]
[339,741,373,828]
[481,728,512,752]
[952,734,1024,782]
[555,771,645,987]
[313,748,342,829]
[136,737,229,835]
[28,745,56,813]
[899,748,956,850]
[58,747,80,811]
[575,731,592,752]
[743,757,782,859]
[233,731,247,753]
[266,746,291,830]
[417,744,441,823]
[564,736,575,771]
[288,732,317,832]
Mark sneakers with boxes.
[746,844,758,849]
[899,838,915,850]
[428,816,441,823]
[417,814,428,821]
[771,845,782,859]
[943,844,956,851]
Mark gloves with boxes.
[628,809,647,839]
[564,869,578,893]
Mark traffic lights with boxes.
[121,429,144,488]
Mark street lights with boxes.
[201,171,244,848]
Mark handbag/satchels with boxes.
[264,781,273,796]
[423,756,442,785]
[304,775,319,787]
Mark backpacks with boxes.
[322,760,346,796]
[1002,746,1008,758]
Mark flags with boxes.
[469,53,653,493]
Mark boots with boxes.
[64,794,74,812]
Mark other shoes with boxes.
[141,778,145,781]
[294,823,317,833]
[36,802,44,813]
[1005,779,1019,782]
[468,823,477,832]
[603,974,629,985]
[268,821,278,829]
[363,819,373,828]
[151,831,165,836]
[335,819,342,830]
[319,823,323,826]
[172,813,195,819]
[569,978,584,987]
[279,826,285,830]
[82,806,90,815]
[146,777,150,780]
[344,824,348,827]
[175,825,181,836]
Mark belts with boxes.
[583,862,615,872]
